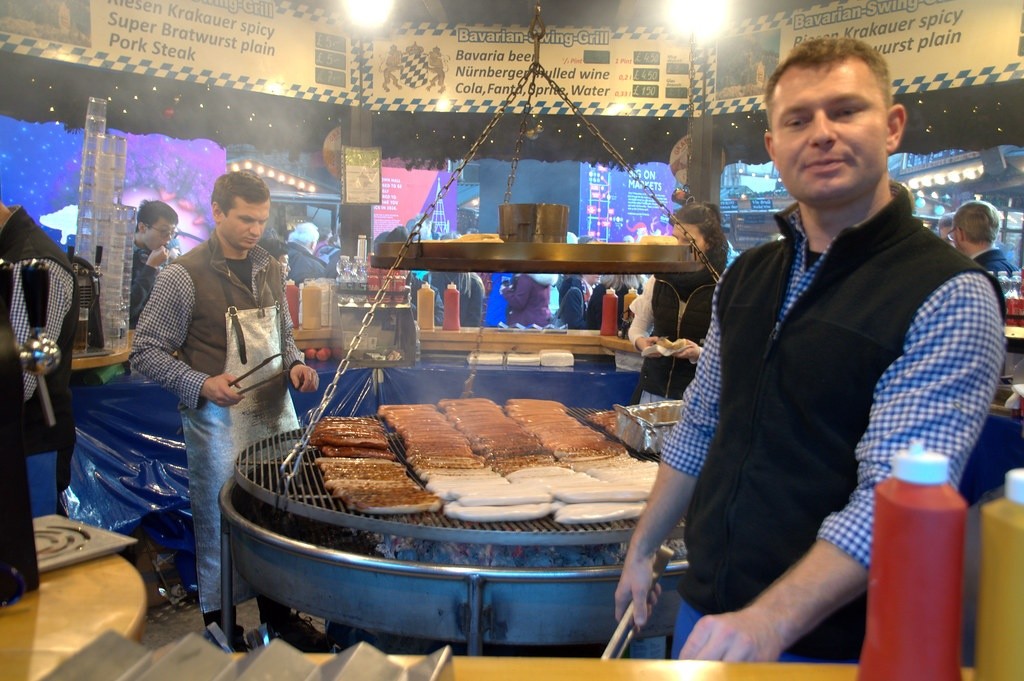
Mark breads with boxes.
[453,233,504,244]
[641,235,678,247]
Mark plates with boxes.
[33,514,137,574]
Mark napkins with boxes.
[467,348,575,366]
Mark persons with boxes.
[1,198,81,522]
[129,170,320,651]
[613,41,1007,664]
[936,200,1019,276]
[129,201,181,331]
[627,200,729,405]
[260,223,670,339]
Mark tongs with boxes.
[226,354,291,394]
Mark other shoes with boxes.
[281,616,329,653]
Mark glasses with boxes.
[947,228,956,242]
[146,223,175,237]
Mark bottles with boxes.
[285,278,300,330]
[300,279,321,329]
[985,268,1022,327]
[976,467,1023,681]
[600,287,619,336]
[623,286,639,331]
[417,282,434,330]
[442,281,460,330]
[335,253,365,282]
[857,437,966,680]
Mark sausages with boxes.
[310,397,659,524]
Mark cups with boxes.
[72,96,138,354]
